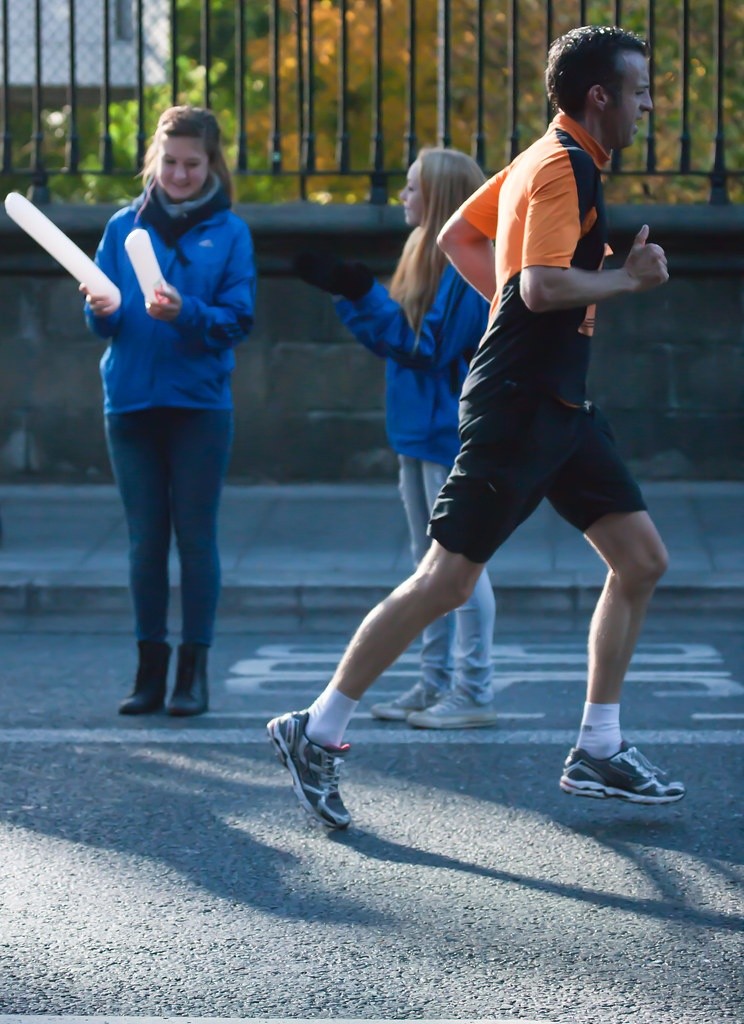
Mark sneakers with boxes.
[370,682,446,720]
[266,710,351,829]
[558,740,686,804]
[406,687,497,729]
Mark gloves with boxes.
[292,253,374,300]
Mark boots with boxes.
[166,642,208,717]
[119,638,171,716]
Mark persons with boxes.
[78,105,258,717]
[266,25,687,830]
[290,148,497,728]
